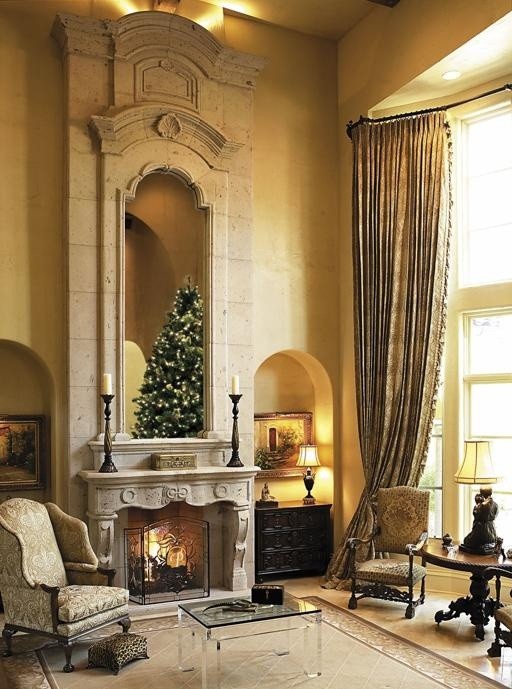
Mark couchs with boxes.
[0,499,133,672]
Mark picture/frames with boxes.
[1,414,51,492]
[255,411,313,480]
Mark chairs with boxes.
[485,563,512,658]
[347,486,428,618]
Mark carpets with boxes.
[0,596,509,688]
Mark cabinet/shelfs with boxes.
[255,499,334,584]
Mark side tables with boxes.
[421,539,511,641]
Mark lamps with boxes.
[296,445,321,503]
[454,440,506,556]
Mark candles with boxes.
[102,371,113,395]
[232,374,241,395]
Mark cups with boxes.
[447,547,455,560]
